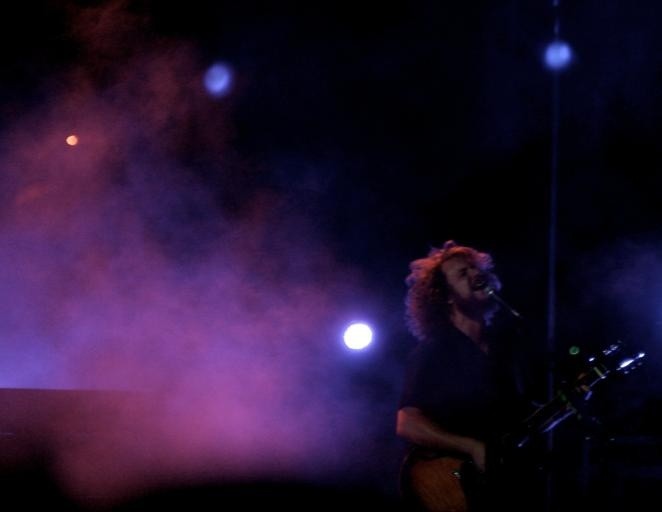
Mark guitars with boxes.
[402,346,645,507]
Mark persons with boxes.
[395,239,549,511]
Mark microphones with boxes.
[485,286,521,319]
